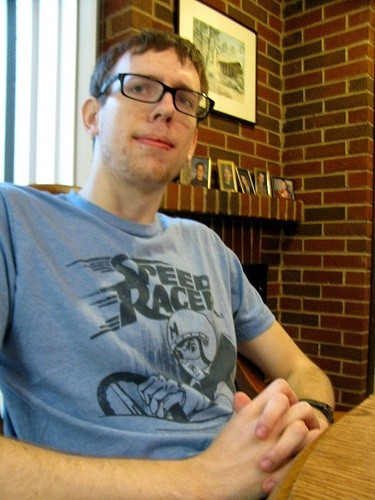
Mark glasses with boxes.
[96,71,215,120]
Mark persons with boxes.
[0,30,336,499]
[256,172,267,194]
[190,162,208,187]
[240,174,254,193]
[221,166,234,189]
[278,180,293,198]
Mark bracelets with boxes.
[296,397,334,426]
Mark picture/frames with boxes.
[174,0,258,125]
[190,155,294,200]
